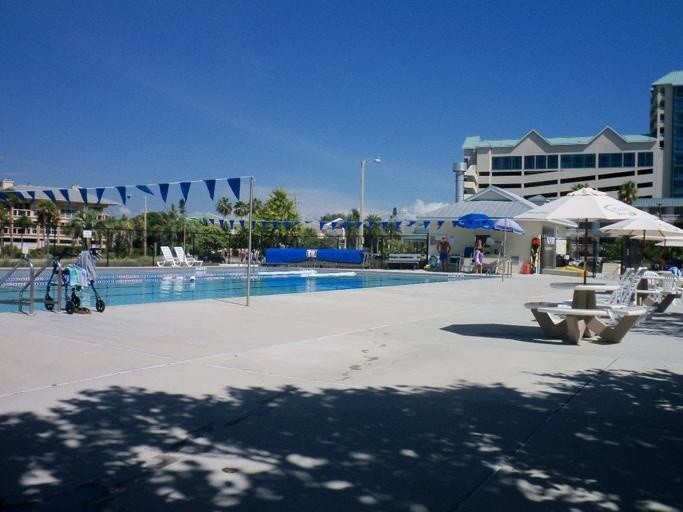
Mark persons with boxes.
[436,236,451,272]
[473,238,486,274]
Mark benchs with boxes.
[524,299,647,346]
[385,253,421,269]
[436,256,461,271]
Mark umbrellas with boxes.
[511,182,660,284]
[493,218,524,261]
[597,214,683,276]
[452,213,496,231]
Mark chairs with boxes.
[156,243,203,267]
[605,265,682,326]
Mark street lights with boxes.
[357,157,381,250]
[125,194,147,255]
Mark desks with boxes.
[549,280,616,337]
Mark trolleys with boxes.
[45,246,106,314]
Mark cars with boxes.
[555,254,604,273]
